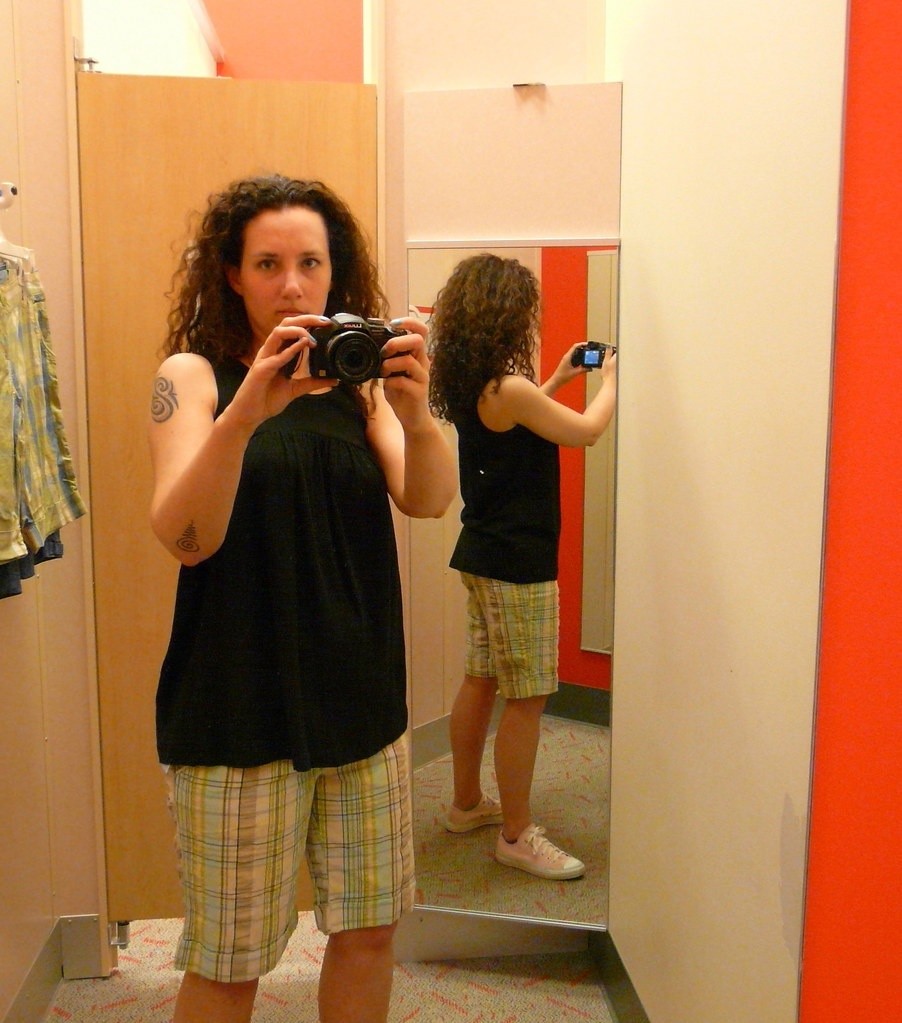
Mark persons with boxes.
[150,174,458,1023]
[425,252,617,882]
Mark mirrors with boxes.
[384,81,623,931]
[582,250,613,653]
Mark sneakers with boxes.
[445,792,504,833]
[495,823,584,879]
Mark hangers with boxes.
[0,182,39,289]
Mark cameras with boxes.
[308,312,409,385]
[582,340,616,367]
